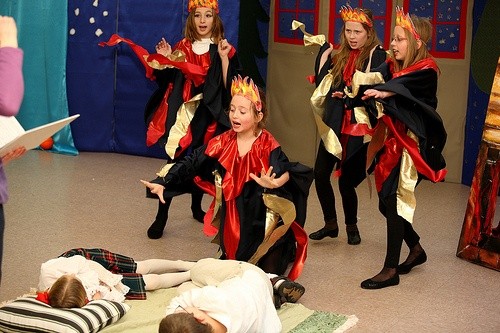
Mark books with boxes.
[0,113,80,158]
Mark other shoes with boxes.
[399,249,427,274]
[308,226,338,240]
[345,225,361,245]
[361,269,399,288]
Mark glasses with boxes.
[174,305,190,315]
[391,35,407,41]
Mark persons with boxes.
[140,74,314,280]
[0,15,25,283]
[159,258,305,333]
[35,247,198,308]
[332,8,449,291]
[292,4,386,246]
[97,0,241,239]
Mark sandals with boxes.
[271,275,305,308]
